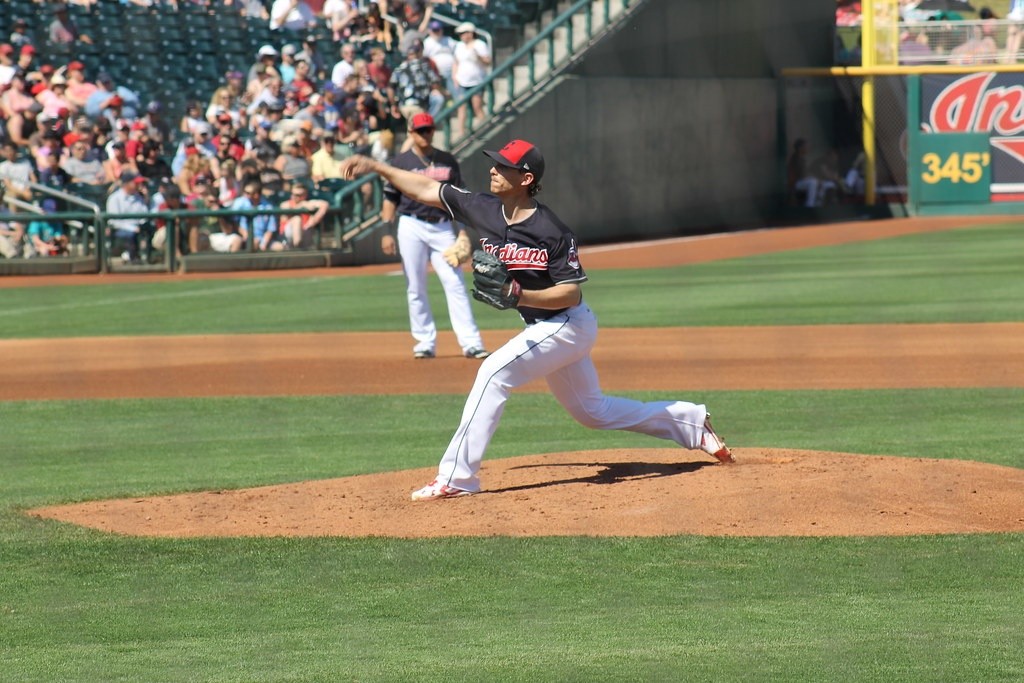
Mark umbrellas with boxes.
[914,0,976,13]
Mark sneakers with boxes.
[412,480,474,502]
[467,347,491,359]
[701,412,737,463]
[414,350,435,358]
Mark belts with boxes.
[402,211,452,223]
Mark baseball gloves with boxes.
[469,249,524,312]
[444,236,474,269]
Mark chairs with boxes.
[0,0,541,229]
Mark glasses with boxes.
[73,146,87,151]
[220,142,230,145]
[295,193,305,196]
[242,191,251,195]
[323,136,335,144]
[195,180,205,184]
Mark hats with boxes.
[20,44,35,54]
[482,139,545,196]
[410,113,436,129]
[259,45,279,61]
[50,74,66,85]
[112,142,125,149]
[96,71,111,83]
[281,44,297,55]
[43,199,57,212]
[218,115,232,122]
[454,21,476,33]
[261,120,272,130]
[147,100,163,113]
[95,116,112,130]
[196,122,212,136]
[159,175,173,186]
[68,61,85,71]
[0,44,14,53]
[120,168,145,183]
[36,112,50,122]
[326,121,338,131]
[116,119,131,130]
[225,71,244,79]
[300,120,313,133]
[110,96,123,107]
[324,82,342,93]
[429,20,442,30]
[130,122,148,130]
[191,174,206,186]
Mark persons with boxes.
[339,140,736,500]
[0,0,494,259]
[381,113,491,359]
[899,0,1024,65]
[790,138,841,207]
